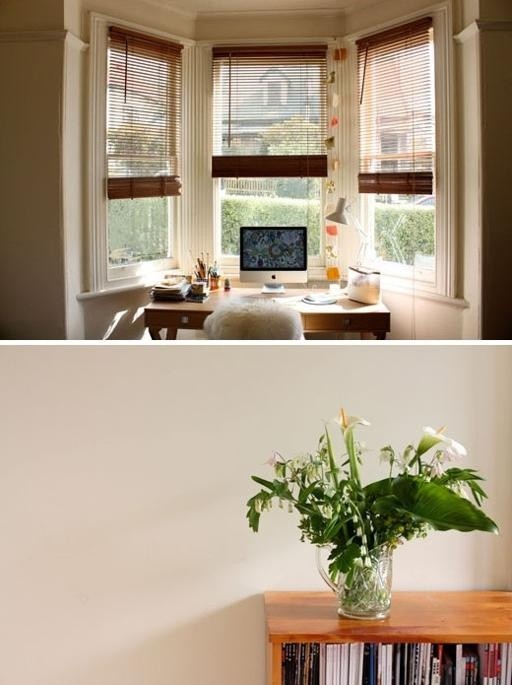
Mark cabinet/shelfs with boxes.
[261,591,511,685]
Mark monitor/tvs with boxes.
[239,227,308,294]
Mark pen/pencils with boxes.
[190,252,221,280]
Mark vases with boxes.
[314,534,406,621]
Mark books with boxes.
[282,641,512,685]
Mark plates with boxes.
[302,296,337,304]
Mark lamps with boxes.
[325,197,371,296]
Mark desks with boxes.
[144,286,391,340]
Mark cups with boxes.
[313,543,395,621]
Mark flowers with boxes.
[246,405,500,610]
